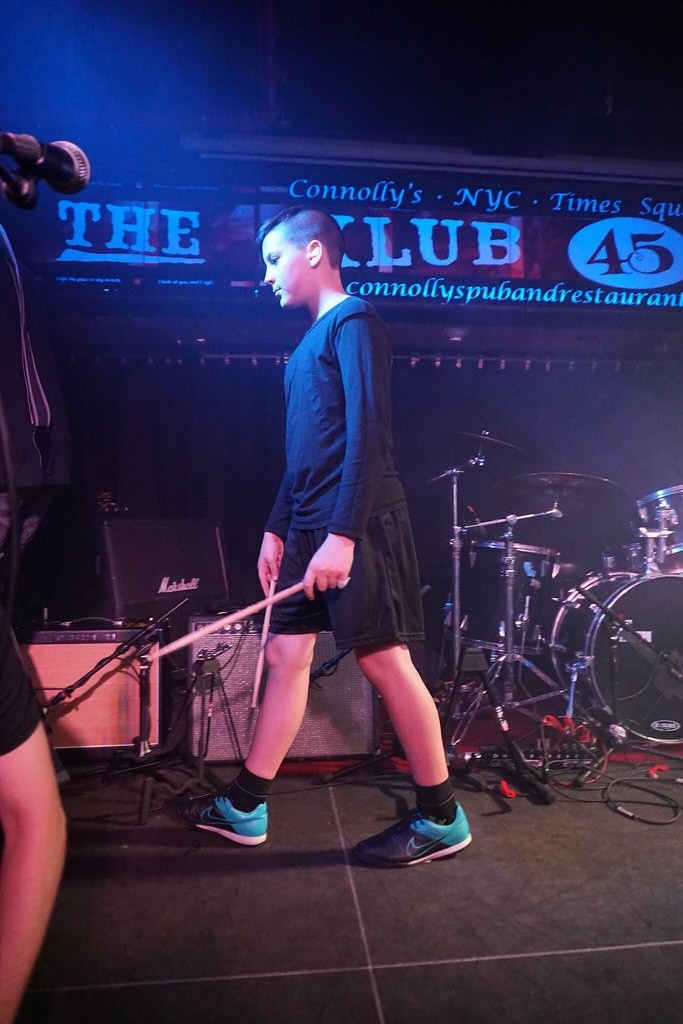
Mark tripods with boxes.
[320,441,683,814]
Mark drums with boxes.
[550,569,683,746]
[445,526,561,656]
[602,481,683,572]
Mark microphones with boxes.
[310,683,323,691]
[0,132,91,195]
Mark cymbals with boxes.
[456,426,525,454]
[492,472,630,517]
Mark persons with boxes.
[179,202,474,867]
[1,600,69,1024]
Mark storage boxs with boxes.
[10,619,166,751]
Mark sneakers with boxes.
[163,784,269,846]
[353,801,472,866]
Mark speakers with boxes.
[16,628,160,749]
[100,516,230,625]
[186,614,381,766]
